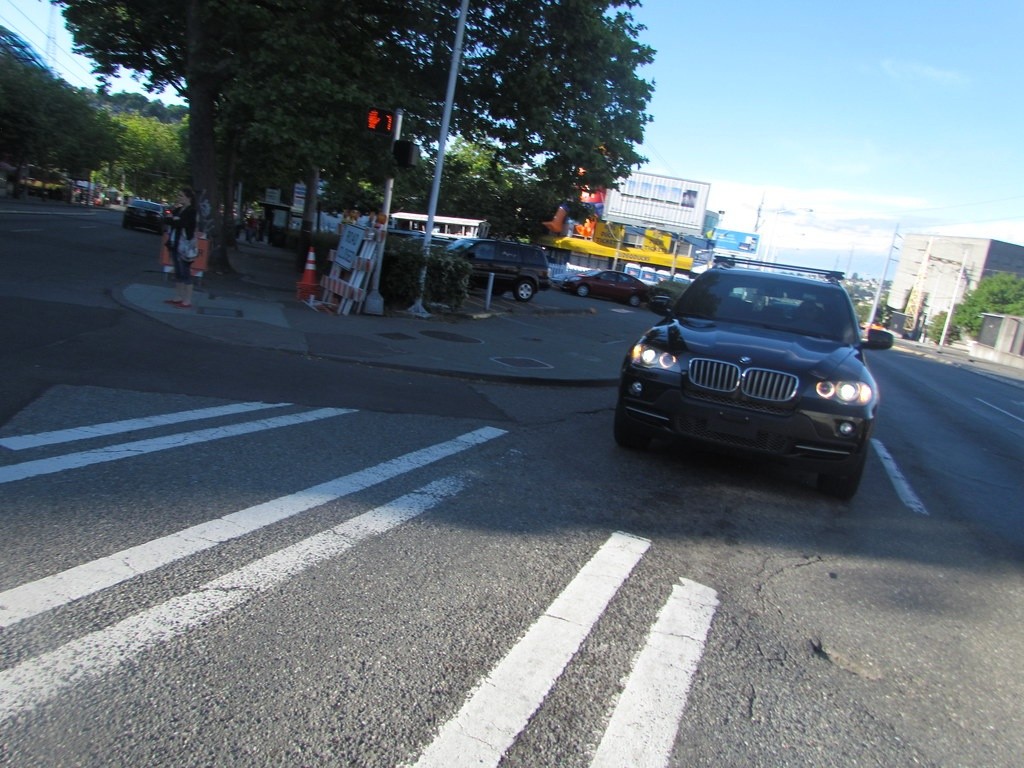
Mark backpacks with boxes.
[177,231,199,262]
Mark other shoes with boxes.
[164,297,185,304]
[176,302,192,308]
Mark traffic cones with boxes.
[296,248,319,299]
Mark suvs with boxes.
[443,238,552,303]
[614,257,893,497]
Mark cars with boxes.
[123,200,174,235]
[559,270,650,307]
[391,230,451,255]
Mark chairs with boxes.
[790,307,825,323]
[714,296,745,318]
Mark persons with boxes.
[794,299,825,331]
[245,214,266,242]
[165,188,199,309]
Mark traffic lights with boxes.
[394,139,418,169]
[367,109,394,135]
